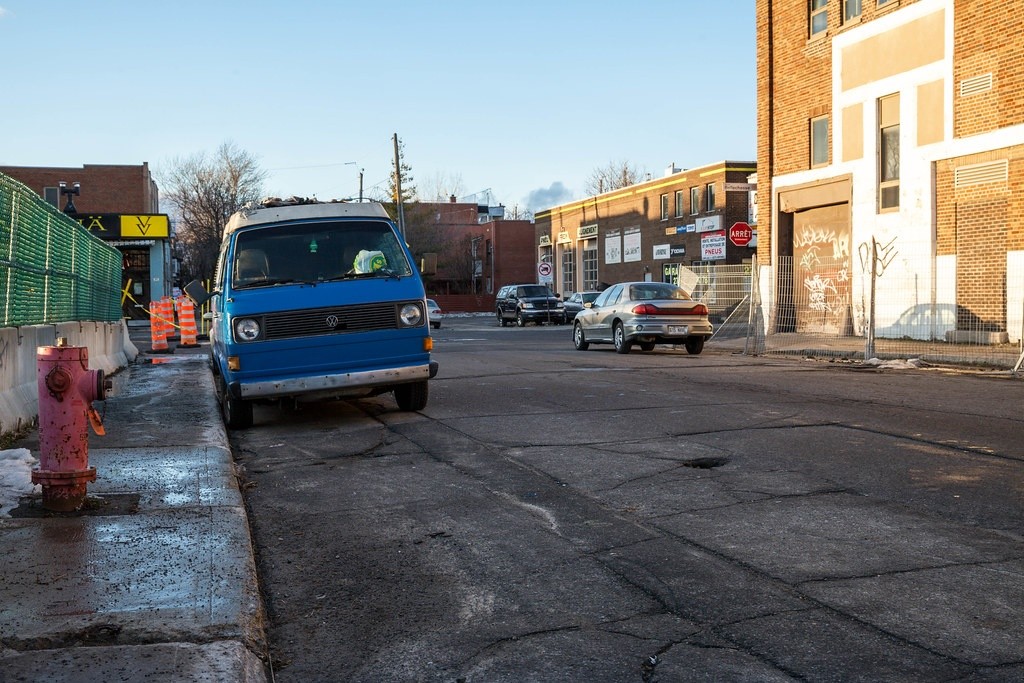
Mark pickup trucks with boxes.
[495,284,567,328]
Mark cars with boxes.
[426,298,441,329]
[562,291,602,325]
[572,282,715,355]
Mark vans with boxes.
[182,198,439,431]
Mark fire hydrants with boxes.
[30,337,113,519]
